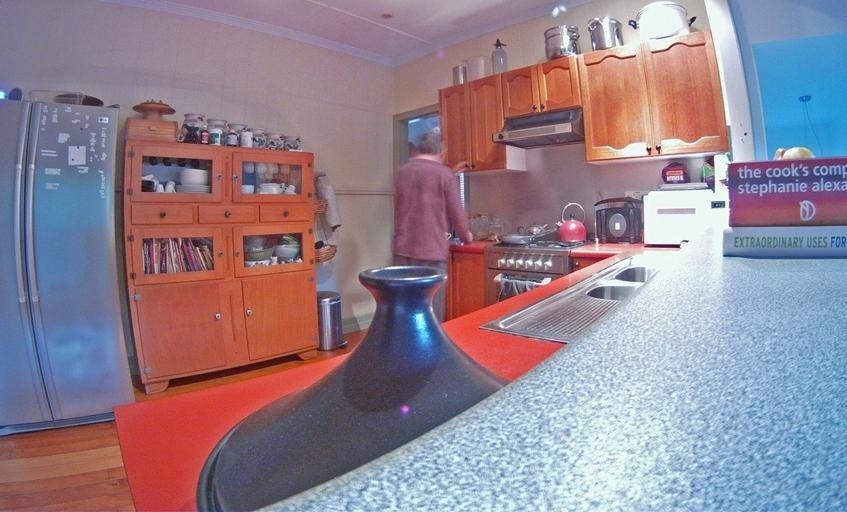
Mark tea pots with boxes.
[517,220,548,235]
[555,201,588,242]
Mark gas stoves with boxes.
[483,232,595,273]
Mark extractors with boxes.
[491,106,585,150]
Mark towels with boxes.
[495,278,535,303]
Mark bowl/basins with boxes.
[180,168,209,186]
[274,245,299,258]
[245,249,274,262]
[242,184,254,193]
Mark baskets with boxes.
[314,199,328,214]
[316,244,337,262]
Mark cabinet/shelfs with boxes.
[574,30,728,165]
[570,255,604,273]
[499,57,582,119]
[437,72,529,176]
[124,141,319,395]
[441,243,485,320]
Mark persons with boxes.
[392,130,474,321]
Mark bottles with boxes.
[180,113,303,152]
[468,215,479,240]
[477,212,489,237]
[194,264,524,512]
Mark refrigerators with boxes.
[0,98,137,437]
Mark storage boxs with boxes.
[724,159,846,228]
[124,116,179,143]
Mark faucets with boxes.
[679,239,689,248]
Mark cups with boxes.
[243,162,291,174]
[464,55,490,79]
[452,65,467,87]
[157,181,176,192]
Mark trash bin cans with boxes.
[318,291,348,351]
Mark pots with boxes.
[544,25,580,58]
[628,1,698,41]
[587,16,624,51]
[500,232,536,244]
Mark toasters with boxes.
[592,195,642,244]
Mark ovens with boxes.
[483,267,562,309]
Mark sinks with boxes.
[614,265,657,284]
[585,285,638,302]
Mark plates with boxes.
[176,184,211,192]
[259,183,281,194]
